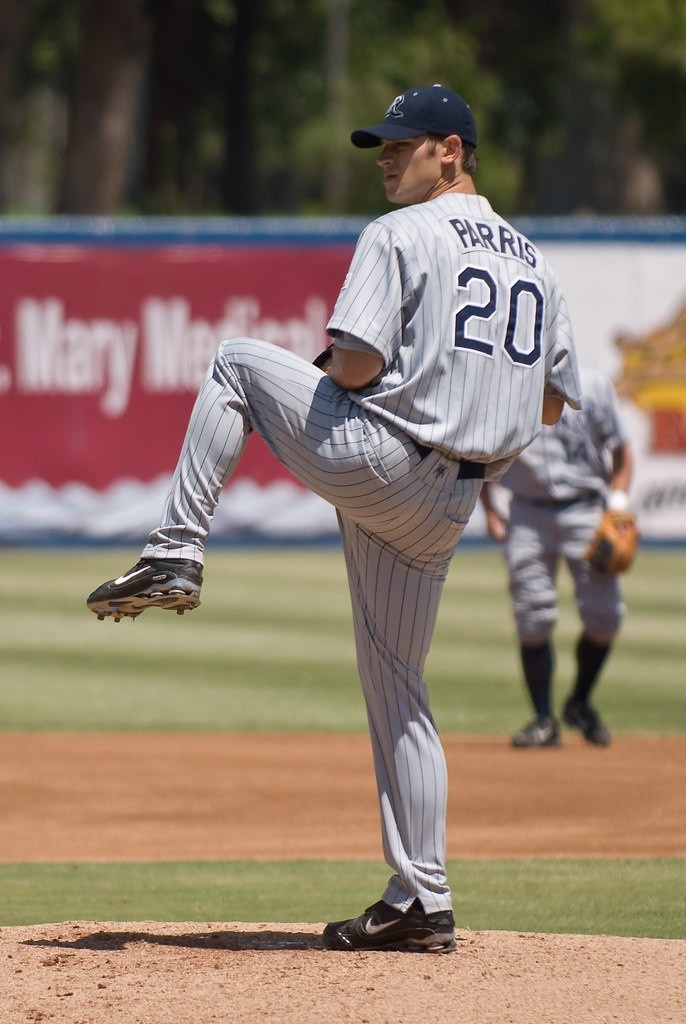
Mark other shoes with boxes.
[512,713,560,746]
[564,699,612,747]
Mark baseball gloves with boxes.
[312,341,334,368]
[584,508,639,576]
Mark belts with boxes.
[532,490,599,510]
[412,439,487,480]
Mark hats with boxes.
[351,84,478,148]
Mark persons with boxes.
[478,364,638,748]
[88,82,585,954]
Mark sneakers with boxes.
[87,556,203,622]
[322,899,457,954]
